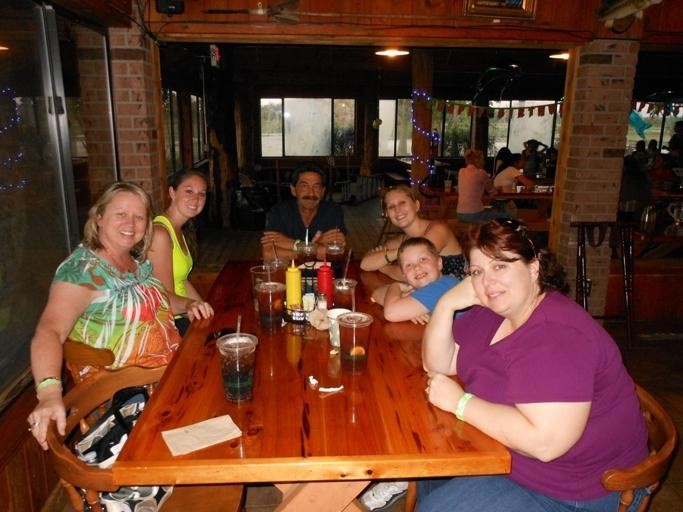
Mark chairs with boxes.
[131,157,683,351]
[601,383,677,512]
[55,338,115,435]
[47,365,168,512]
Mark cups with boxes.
[326,309,351,348]
[249,241,357,335]
[515,184,554,193]
[444,180,452,193]
[215,333,259,404]
[336,312,373,375]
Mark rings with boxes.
[26,425,32,433]
[31,421,40,427]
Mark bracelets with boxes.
[293,238,301,252]
[33,375,62,392]
[453,392,476,422]
[381,247,391,266]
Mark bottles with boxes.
[640,205,656,224]
[286,332,303,369]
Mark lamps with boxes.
[156,0,185,18]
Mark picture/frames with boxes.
[462,0,538,23]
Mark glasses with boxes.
[500,218,536,260]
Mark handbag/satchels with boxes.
[74,386,173,512]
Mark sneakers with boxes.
[360,482,409,512]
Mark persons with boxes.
[144,168,215,336]
[26,179,184,512]
[455,120,557,229]
[617,120,682,240]
[260,163,350,262]
[370,238,461,325]
[410,219,650,512]
[359,184,470,284]
